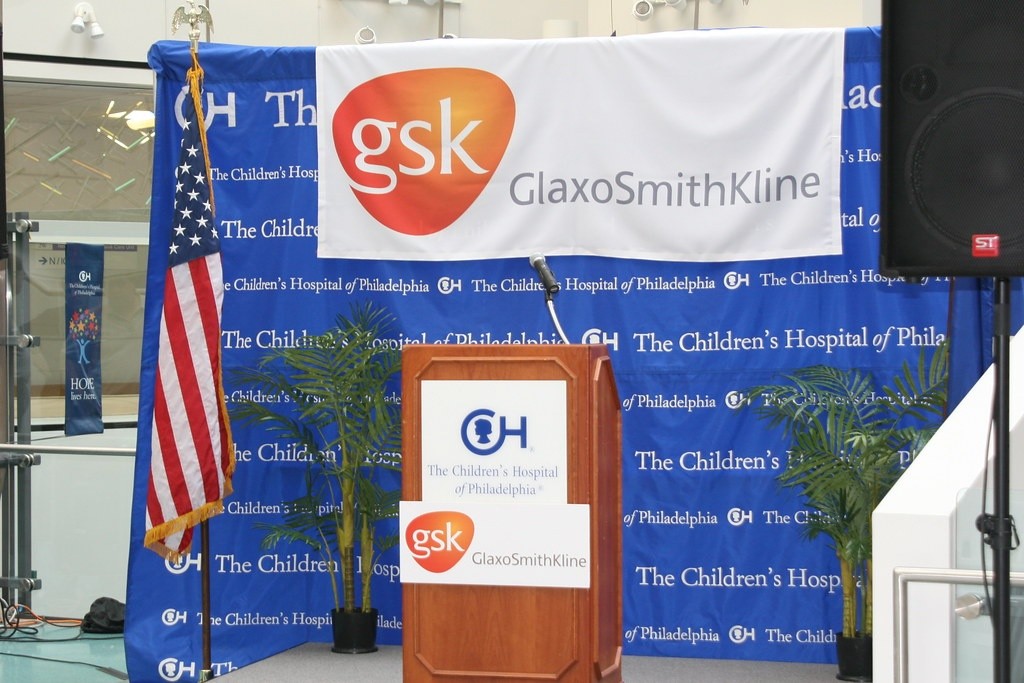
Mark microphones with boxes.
[529,253,560,294]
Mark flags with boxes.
[144,48,234,565]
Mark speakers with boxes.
[879,0,1024,277]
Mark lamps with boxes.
[71,2,104,39]
[632,0,687,23]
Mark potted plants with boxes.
[229,298,401,649]
[733,337,950,679]
